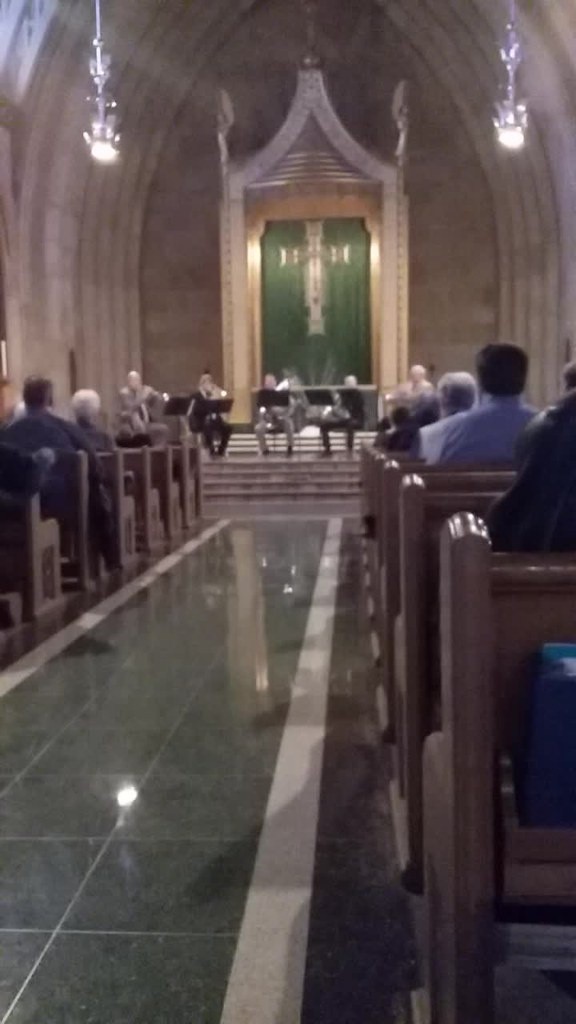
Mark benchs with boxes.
[358,444,576,1024]
[0,432,204,628]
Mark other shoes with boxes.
[317,449,331,458]
[257,449,269,457]
[285,445,293,457]
[208,450,217,459]
[218,447,227,458]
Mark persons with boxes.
[375,344,576,556]
[254,373,294,456]
[0,371,234,584]
[317,375,365,459]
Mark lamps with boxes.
[491,0,530,148]
[82,0,123,161]
